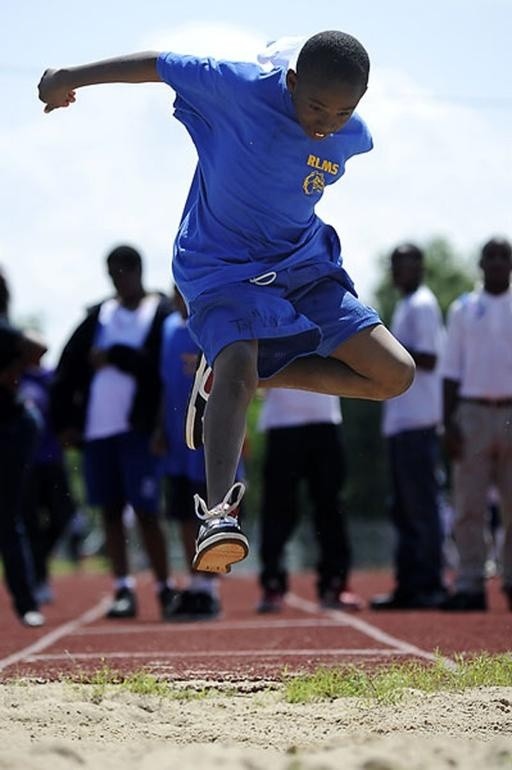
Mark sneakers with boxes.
[186,349,214,450]
[256,589,282,613]
[108,586,136,617]
[158,587,220,621]
[192,482,249,576]
[17,603,45,626]
[35,584,53,607]
[320,584,487,611]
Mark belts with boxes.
[479,399,510,406]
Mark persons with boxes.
[36,31,416,577]
[438,238,511,618]
[49,250,174,620]
[257,386,352,616]
[0,272,68,629]
[155,310,251,620]
[371,246,450,613]
